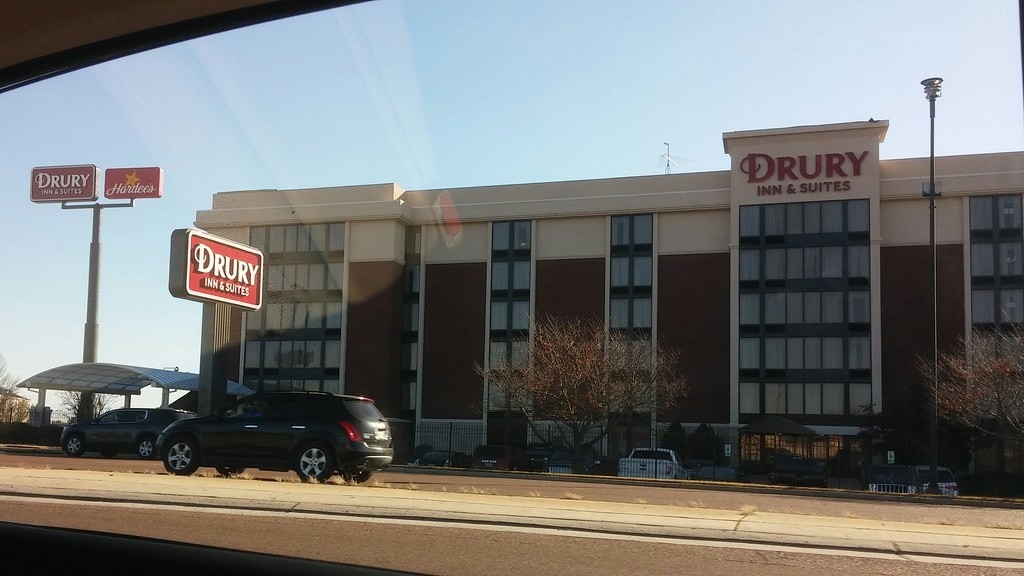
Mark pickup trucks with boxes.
[617,449,693,480]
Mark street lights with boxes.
[920,77,945,492]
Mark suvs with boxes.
[863,464,918,495]
[533,446,606,475]
[156,389,395,483]
[469,444,530,473]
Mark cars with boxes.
[699,458,778,484]
[59,407,200,459]
[915,465,960,497]
[408,450,470,469]
